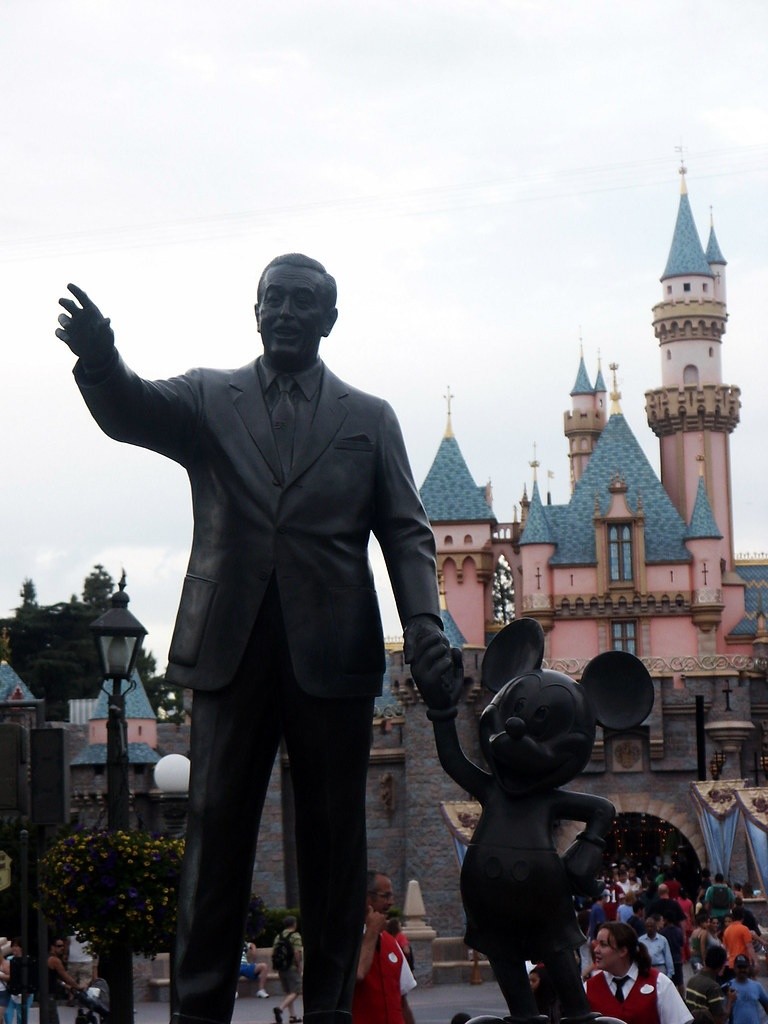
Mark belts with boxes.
[652,964,665,967]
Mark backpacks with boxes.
[271,931,298,971]
[713,886,730,910]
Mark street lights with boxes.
[86,575,150,1024]
[153,752,191,1020]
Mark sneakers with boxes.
[234,991,240,1000]
[256,989,269,998]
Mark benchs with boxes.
[150,947,303,1002]
[432,937,495,984]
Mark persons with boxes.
[526,857,768,1024]
[235,942,269,999]
[352,869,418,1024]
[55,254,452,1024]
[0,936,88,1024]
[271,916,303,1024]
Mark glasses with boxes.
[51,944,65,948]
[368,890,391,897]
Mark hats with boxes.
[735,954,750,968]
[0,937,11,955]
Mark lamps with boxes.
[708,746,768,779]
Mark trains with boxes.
[68,978,108,1024]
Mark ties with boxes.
[613,975,630,1002]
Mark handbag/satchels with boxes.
[5,985,10,992]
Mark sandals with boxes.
[273,1006,282,1023]
[288,1015,303,1024]
